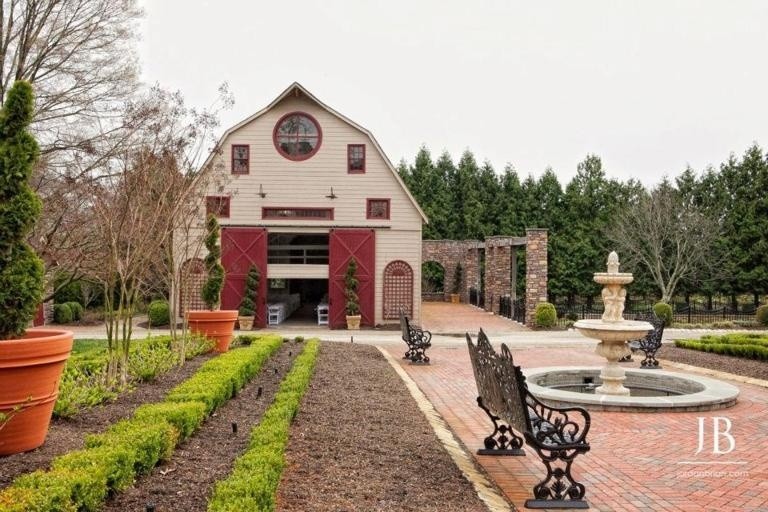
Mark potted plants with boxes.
[0,81,74,454]
[420,261,444,301]
[450,261,462,304]
[185,215,239,352]
[238,265,259,330]
[344,257,362,329]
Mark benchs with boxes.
[617,309,665,370]
[398,307,432,365]
[465,327,591,509]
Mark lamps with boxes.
[325,187,338,201]
[255,184,267,198]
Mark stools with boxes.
[314,294,329,325]
[267,293,300,325]
